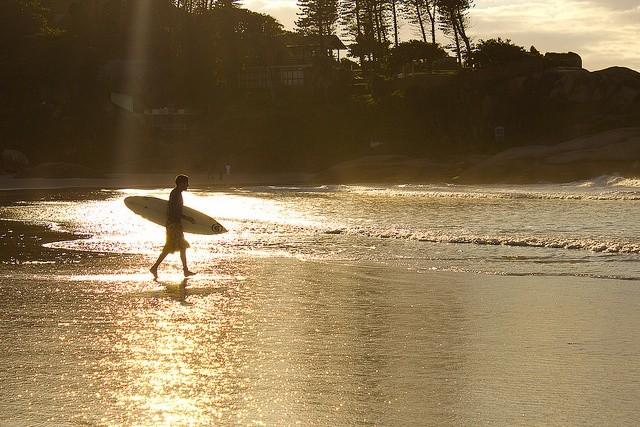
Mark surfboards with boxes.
[124,195,228,234]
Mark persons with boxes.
[147,173,199,281]
